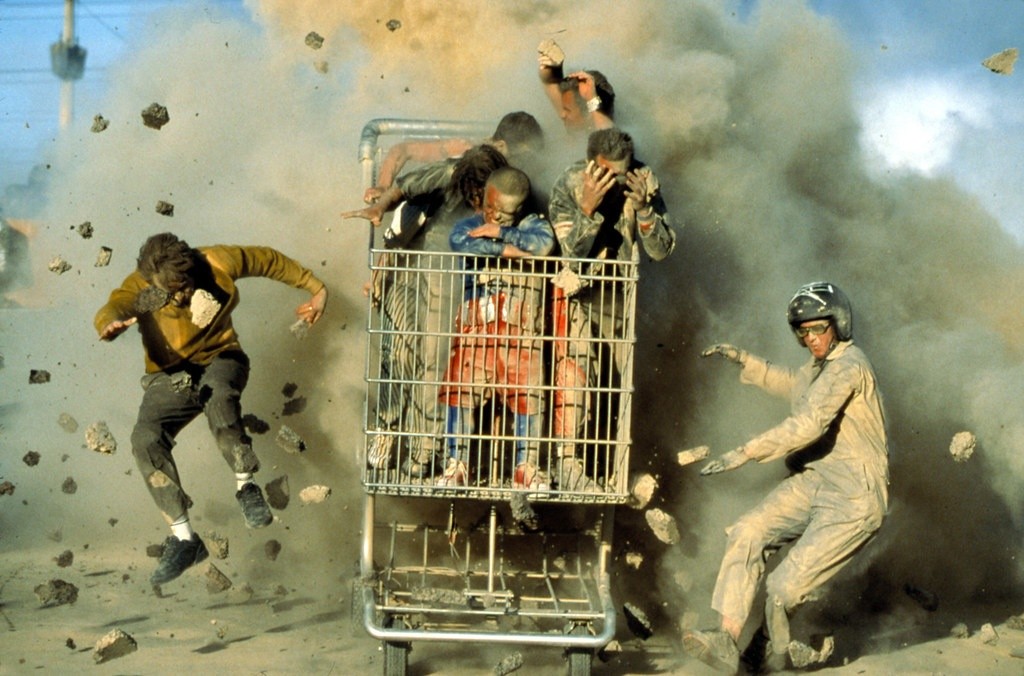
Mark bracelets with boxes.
[637,206,656,228]
[586,96,601,108]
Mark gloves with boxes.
[701,343,747,365]
[698,448,746,476]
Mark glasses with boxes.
[794,320,831,338]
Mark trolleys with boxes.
[358,118,641,676]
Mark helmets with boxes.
[786,281,853,348]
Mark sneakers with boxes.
[681,628,740,675]
[555,459,604,499]
[235,482,273,529]
[512,464,551,499]
[436,459,473,494]
[368,427,399,470]
[404,448,432,478]
[150,532,209,583]
[744,631,787,670]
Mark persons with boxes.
[682,281,892,674]
[95,234,328,586]
[340,112,544,481]
[436,167,555,494]
[548,126,675,492]
[534,37,616,151]
